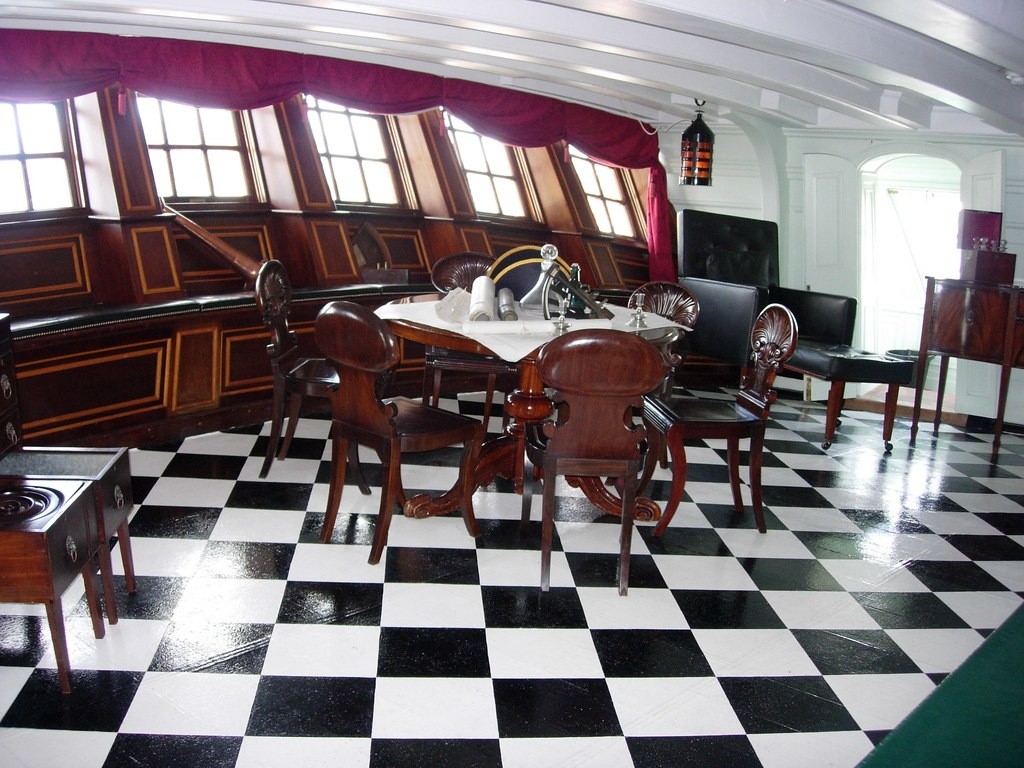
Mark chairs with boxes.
[419,251,520,428]
[253,259,395,495]
[635,303,800,538]
[522,328,666,596]
[627,278,702,369]
[675,209,915,453]
[314,301,485,563]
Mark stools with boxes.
[781,341,914,450]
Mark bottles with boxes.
[567,263,586,309]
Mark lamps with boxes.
[678,98,716,185]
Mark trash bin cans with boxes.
[886,349,935,389]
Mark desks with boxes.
[0,447,139,695]
[379,292,686,523]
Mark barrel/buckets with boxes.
[886,349,934,388]
